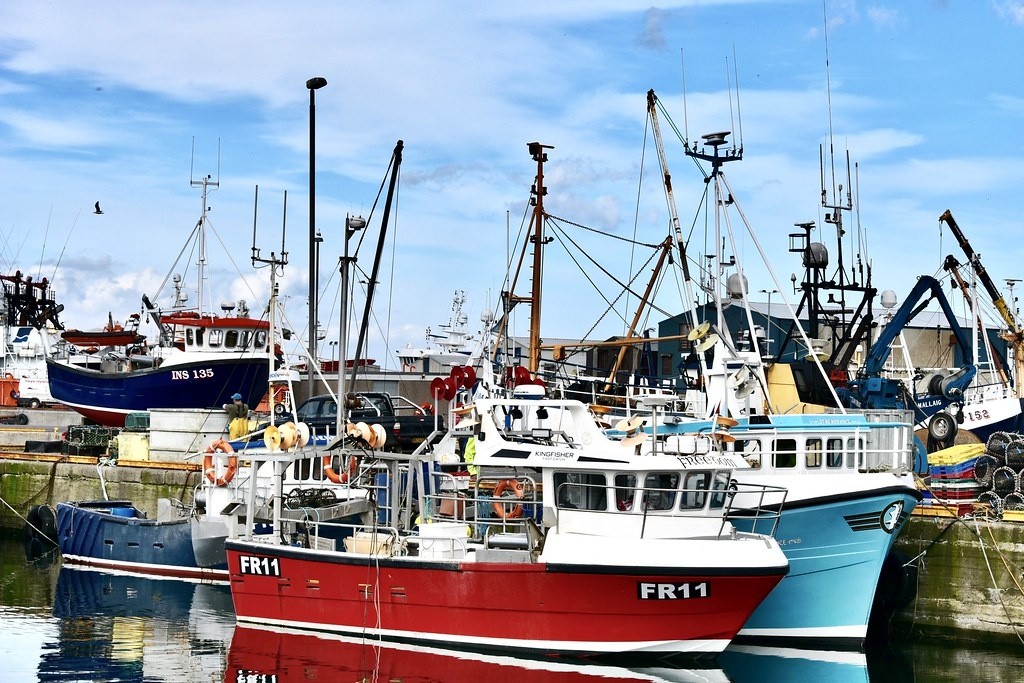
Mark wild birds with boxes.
[93,201,104,215]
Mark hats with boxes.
[231,393,242,399]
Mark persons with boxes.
[223,393,248,441]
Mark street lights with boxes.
[306,77,328,396]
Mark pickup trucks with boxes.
[251,392,444,455]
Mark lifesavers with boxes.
[492,479,524,517]
[204,440,238,488]
[415,403,435,415]
[25,504,57,542]
[322,453,356,484]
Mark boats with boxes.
[224,621,731,683]
[716,644,870,683]
[0,0,1024,656]
